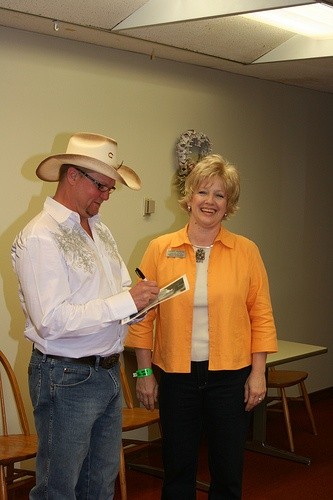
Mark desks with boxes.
[125,339,328,494]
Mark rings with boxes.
[258,398,262,401]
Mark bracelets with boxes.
[133,368,153,378]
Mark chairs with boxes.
[118,359,160,500]
[0,350,38,500]
[265,367,317,452]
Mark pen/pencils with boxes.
[134,267,148,281]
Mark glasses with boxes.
[74,165,116,194]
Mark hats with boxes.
[35,134,142,191]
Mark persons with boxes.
[12,131,159,500]
[129,154,277,500]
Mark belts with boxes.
[36,349,120,369]
